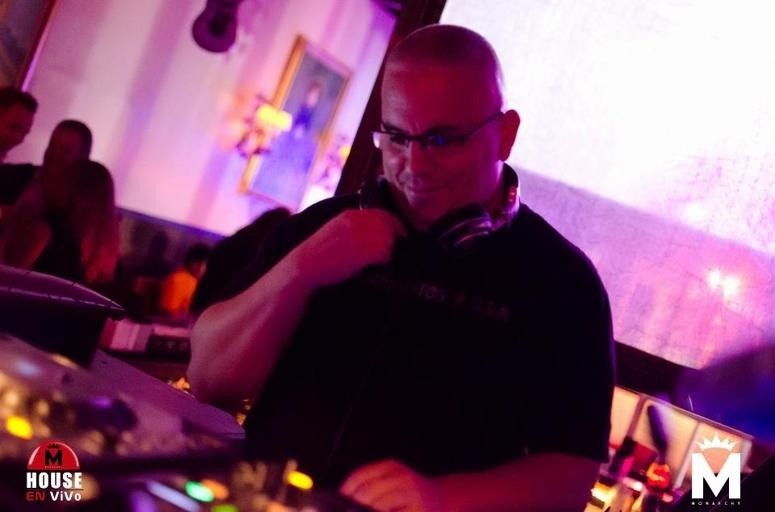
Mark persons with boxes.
[0,160,120,289]
[157,243,210,324]
[186,22,619,511]
[1,115,91,205]
[0,84,39,158]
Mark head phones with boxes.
[358,162,518,250]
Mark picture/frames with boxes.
[240,36,354,214]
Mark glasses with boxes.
[370,110,504,157]
